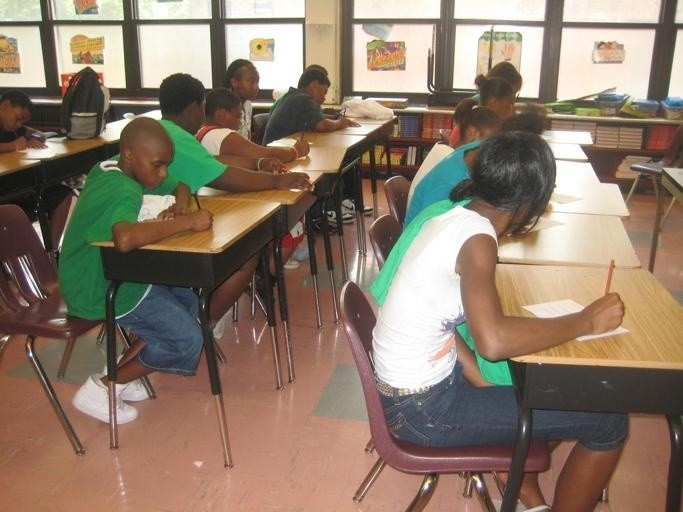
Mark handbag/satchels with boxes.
[56,67,105,140]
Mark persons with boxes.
[196,58,372,299]
[58,117,215,424]
[144,72,314,338]
[373,130,627,512]
[379,61,545,386]
[0,90,72,264]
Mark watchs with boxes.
[28,133,43,142]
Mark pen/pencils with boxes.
[12,127,20,138]
[192,193,202,211]
[605,261,615,295]
[343,108,346,117]
[299,121,308,140]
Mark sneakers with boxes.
[73,375,137,425]
[307,219,337,235]
[327,209,356,225]
[284,259,299,270]
[341,199,373,216]
[99,363,150,401]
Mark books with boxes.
[614,121,674,179]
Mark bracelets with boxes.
[11,142,19,151]
